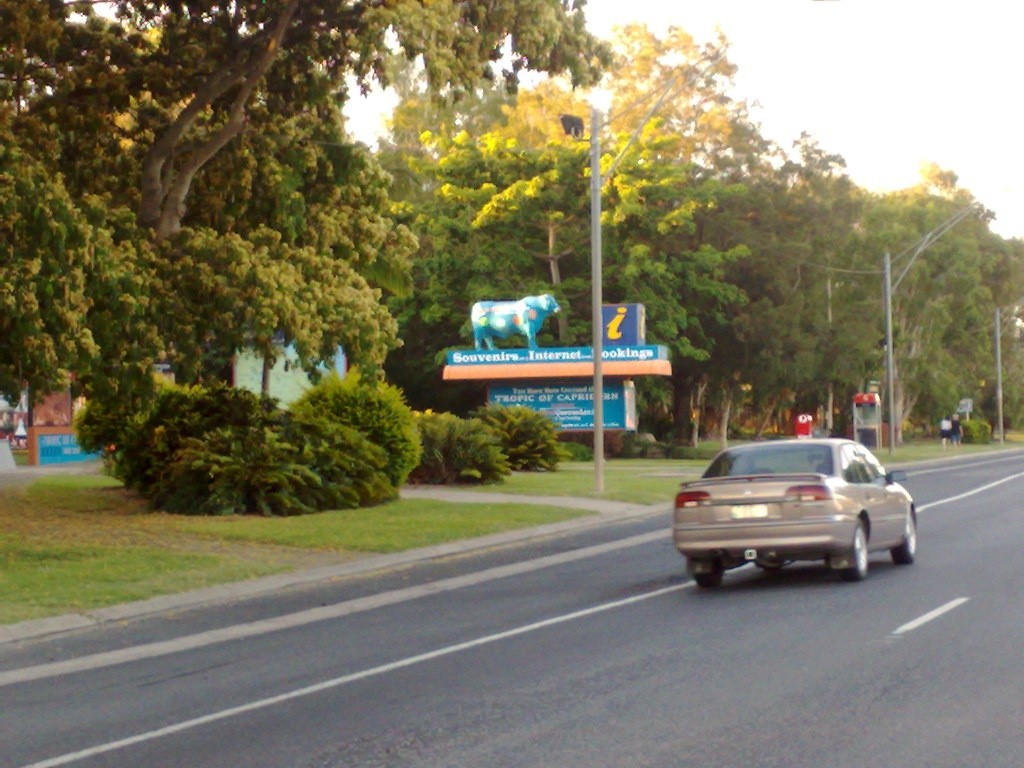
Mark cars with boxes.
[674,436,919,587]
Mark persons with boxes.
[940,415,952,448]
[951,414,964,449]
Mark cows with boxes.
[471,294,561,350]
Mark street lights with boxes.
[560,104,607,496]
[883,193,994,455]
